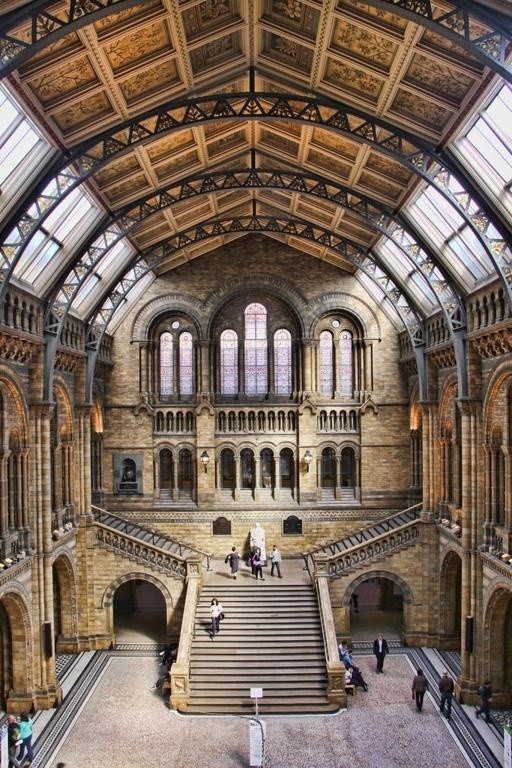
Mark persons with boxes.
[252,548,264,581]
[210,597,224,641]
[251,522,264,558]
[8,714,23,768]
[436,670,455,722]
[269,544,283,578]
[410,668,430,713]
[17,711,34,762]
[153,641,179,689]
[226,545,241,578]
[372,634,389,674]
[473,679,494,724]
[339,638,368,693]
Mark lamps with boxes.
[303,452,313,473]
[200,451,209,474]
